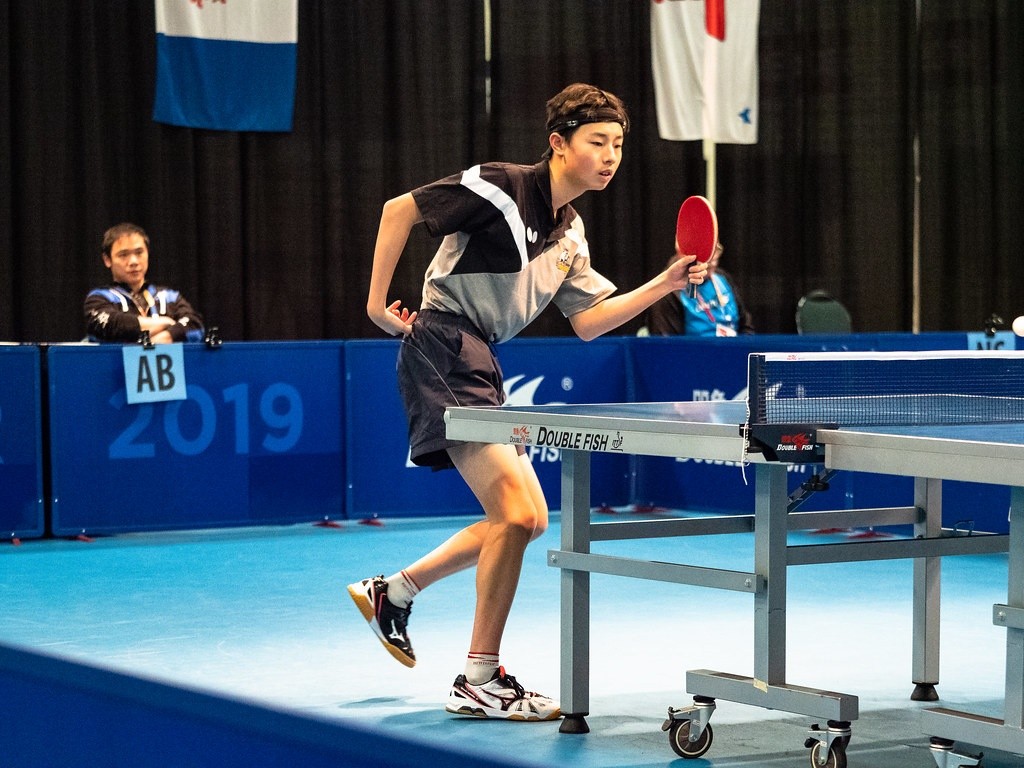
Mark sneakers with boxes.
[347,574,417,668]
[446,666,561,721]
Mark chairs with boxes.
[795,290,854,334]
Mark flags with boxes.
[153,0,298,131]
[652,0,761,145]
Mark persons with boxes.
[344,82,709,721]
[83,222,207,345]
[646,236,754,337]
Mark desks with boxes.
[444,350,1024,768]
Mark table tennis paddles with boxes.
[676,196,718,298]
